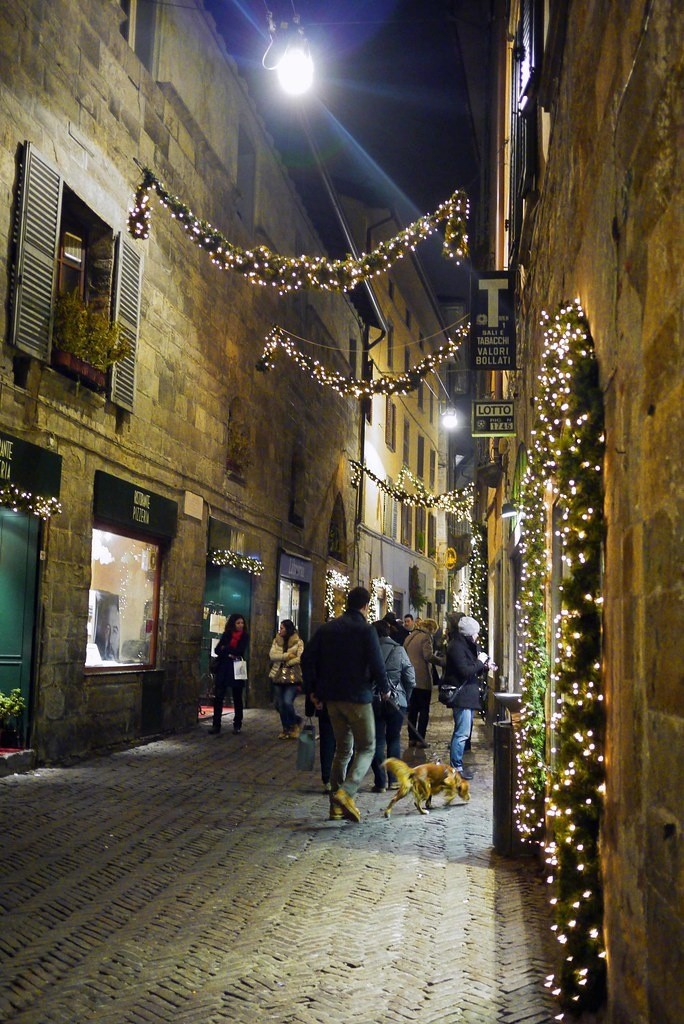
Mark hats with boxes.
[458,617,480,636]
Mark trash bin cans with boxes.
[491,692,523,857]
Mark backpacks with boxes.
[371,645,402,717]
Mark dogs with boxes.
[380,758,472,818]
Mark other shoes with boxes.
[458,771,472,780]
[209,727,220,734]
[233,729,240,734]
[325,781,331,792]
[409,739,431,748]
[290,720,306,738]
[330,806,360,820]
[333,789,361,823]
[372,785,386,793]
[389,782,401,789]
[448,743,471,750]
[279,732,290,740]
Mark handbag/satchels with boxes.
[297,717,315,771]
[234,656,247,680]
[278,665,298,686]
[439,685,459,705]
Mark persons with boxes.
[444,612,498,780]
[433,626,448,651]
[403,618,444,748]
[302,587,391,822]
[270,620,305,739]
[382,612,414,646]
[99,599,119,660]
[372,620,417,795]
[208,613,250,735]
